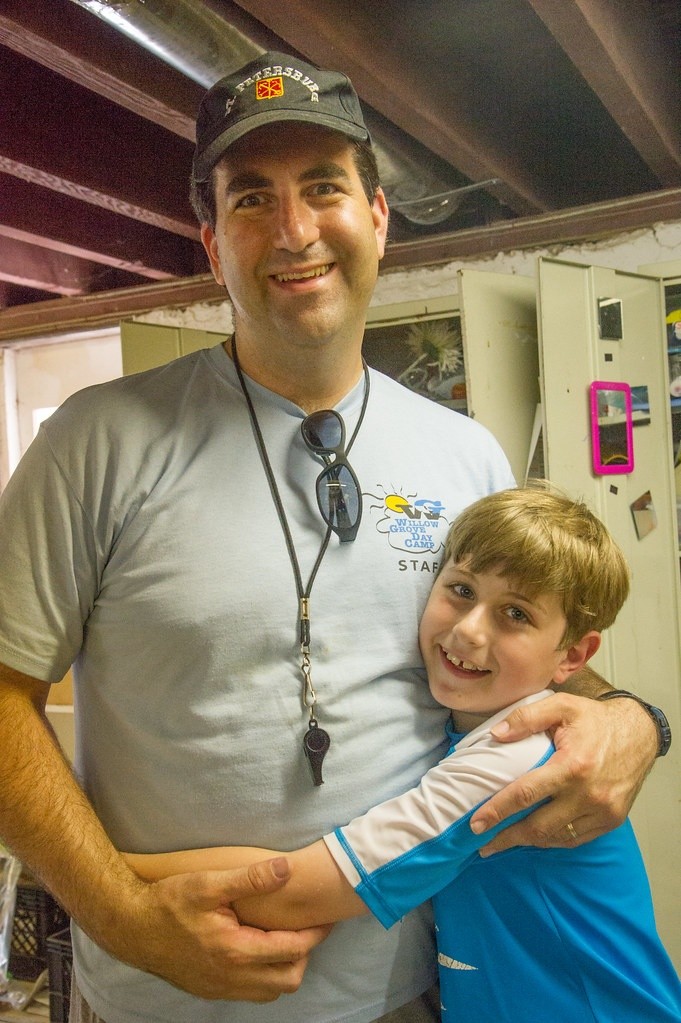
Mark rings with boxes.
[567,821,582,839]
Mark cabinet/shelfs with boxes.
[118,254,680,979]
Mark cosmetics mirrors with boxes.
[589,376,638,478]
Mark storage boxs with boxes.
[46,927,72,1022]
[7,884,70,982]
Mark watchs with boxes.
[597,688,672,761]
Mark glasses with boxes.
[301,408,362,542]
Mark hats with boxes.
[192,50,372,183]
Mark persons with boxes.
[101,482,679,1022]
[1,51,678,1019]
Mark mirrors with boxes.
[590,381,634,475]
